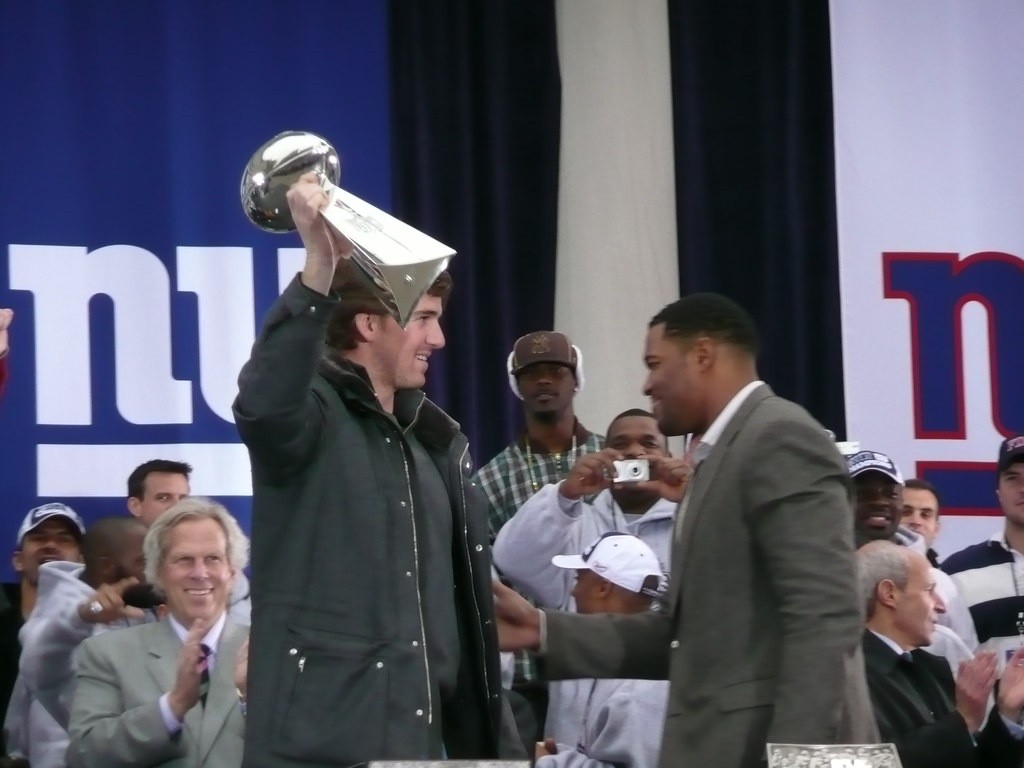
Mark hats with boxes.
[997,433,1024,470]
[16,502,85,550]
[507,330,584,399]
[552,531,664,597]
[845,451,903,484]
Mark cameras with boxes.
[611,458,650,483]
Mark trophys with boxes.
[240,131,457,331]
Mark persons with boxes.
[232,172,503,768]
[492,295,881,768]
[0,331,1024,768]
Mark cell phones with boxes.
[122,584,165,610]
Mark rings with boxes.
[89,601,104,614]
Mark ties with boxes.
[196,643,211,708]
[902,650,914,663]
[675,443,707,544]
